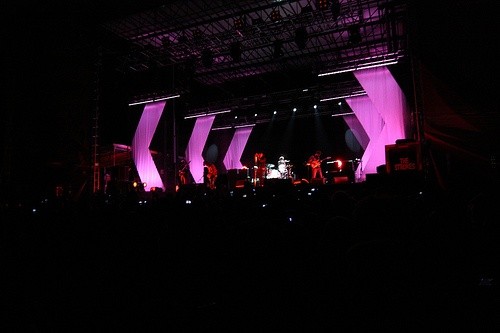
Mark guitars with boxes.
[310,156,332,168]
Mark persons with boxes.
[203,161,217,190]
[258,153,267,185]
[178,159,189,186]
[278,156,291,179]
[307,150,324,179]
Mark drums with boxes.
[278,164,286,172]
[266,169,282,179]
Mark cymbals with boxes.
[267,164,275,167]
[278,160,290,162]
